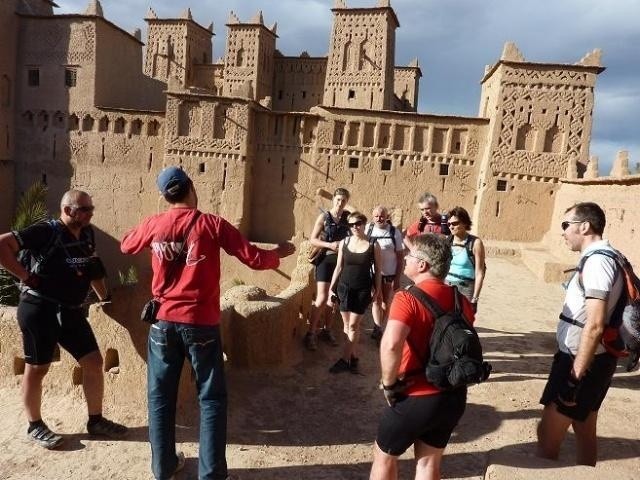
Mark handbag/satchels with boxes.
[306,230,328,266]
[140,299,160,323]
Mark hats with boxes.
[156,166,188,197]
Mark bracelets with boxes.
[380,376,400,392]
[100,296,111,303]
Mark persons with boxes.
[302,189,486,374]
[0,189,130,451]
[120,167,296,480]
[368,237,476,480]
[537,202,628,468]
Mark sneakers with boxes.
[304,333,318,352]
[27,423,65,448]
[371,327,382,347]
[328,356,359,374]
[169,451,186,480]
[87,417,129,439]
[319,328,339,347]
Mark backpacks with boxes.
[404,283,492,389]
[577,249,640,372]
[18,219,61,291]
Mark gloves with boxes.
[381,377,406,407]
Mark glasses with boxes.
[447,221,461,226]
[561,221,584,231]
[349,221,364,227]
[69,206,94,213]
